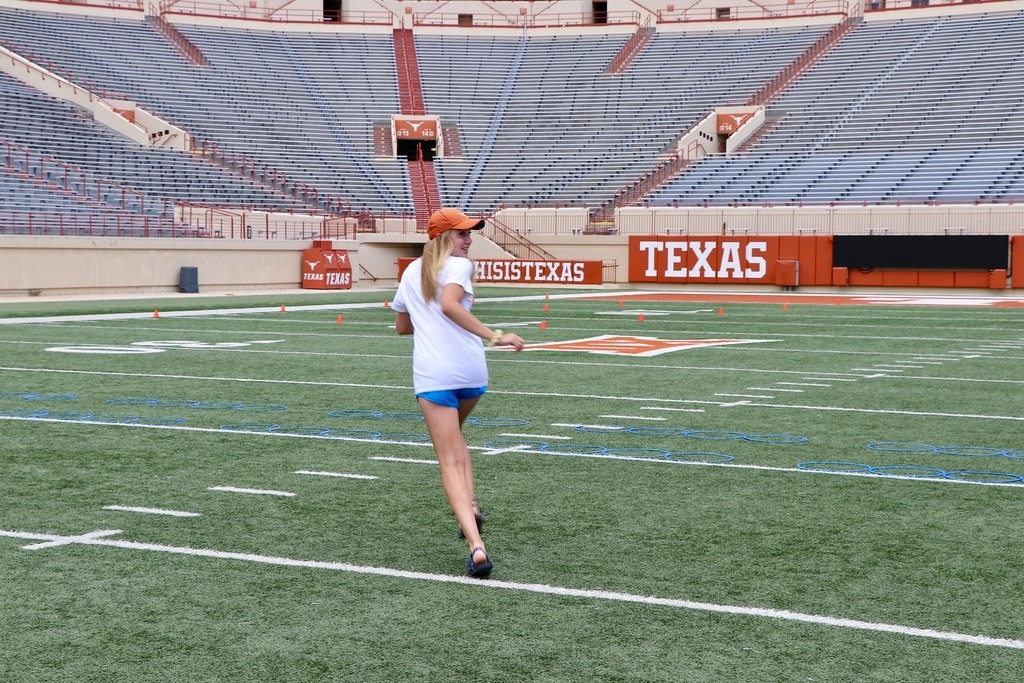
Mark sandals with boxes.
[459,503,483,538]
[468,548,493,577]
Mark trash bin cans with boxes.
[178,266,200,293]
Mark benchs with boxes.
[0,3,1024,236]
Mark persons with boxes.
[390,208,524,575]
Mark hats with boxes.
[427,208,485,240]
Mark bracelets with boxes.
[487,329,504,347]
